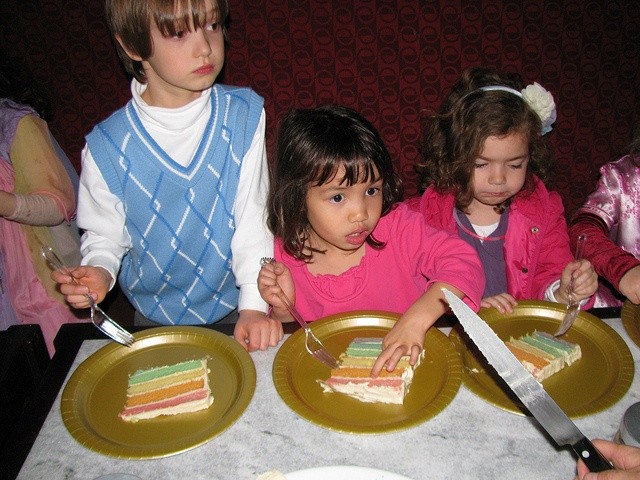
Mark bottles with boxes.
[614,402,639,448]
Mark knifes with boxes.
[440,286,615,473]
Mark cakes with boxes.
[504,328,583,382]
[120,358,215,424]
[315,337,426,405]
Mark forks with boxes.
[259,257,340,370]
[554,233,588,338]
[40,244,136,345]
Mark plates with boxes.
[621,299,640,347]
[272,310,463,435]
[448,299,635,419]
[60,324,257,461]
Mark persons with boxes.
[568,138,640,309]
[257,104,486,378]
[574,438,640,480]
[399,63,600,314]
[51,0,284,352]
[1,98,95,360]
